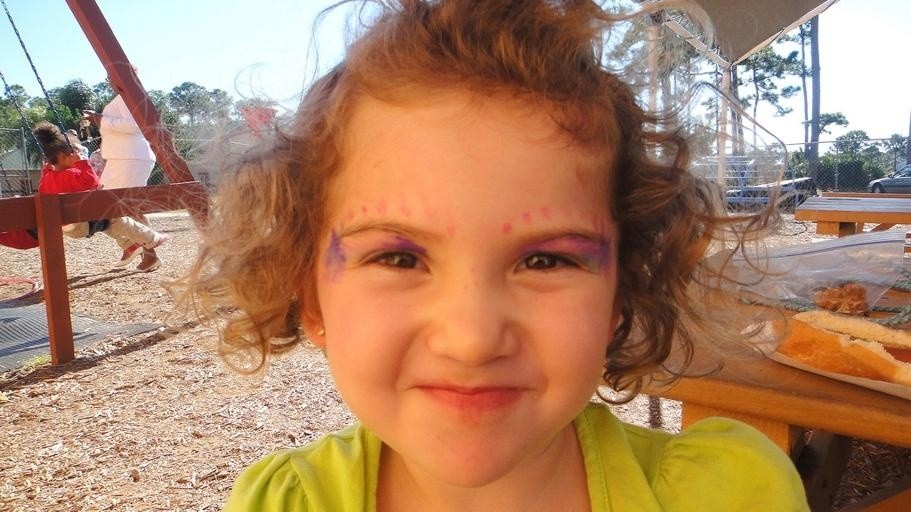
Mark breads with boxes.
[774,310,911,387]
[814,285,867,314]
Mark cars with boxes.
[869,163,911,194]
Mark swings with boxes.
[0,0,108,249]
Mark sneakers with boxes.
[134,255,164,273]
[114,243,143,268]
[144,231,168,250]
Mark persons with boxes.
[89,136,106,177]
[160,1,811,512]
[31,123,168,257]
[83,64,162,273]
